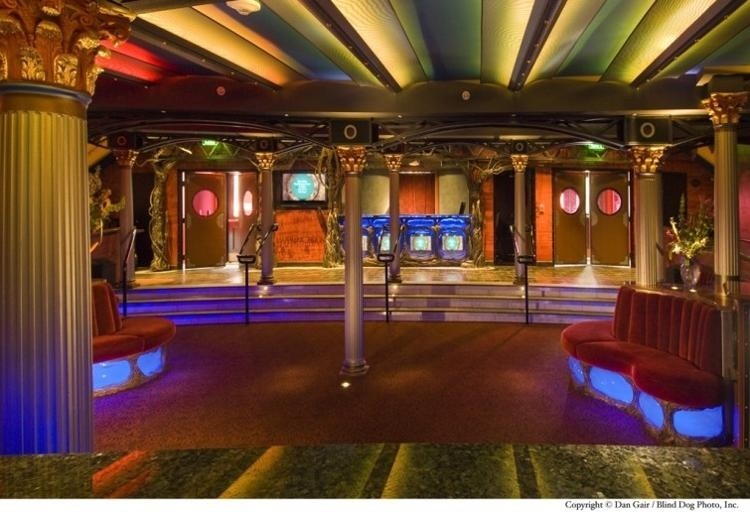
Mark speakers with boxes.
[625,115,673,145]
[329,118,378,144]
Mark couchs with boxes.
[559,286,737,449]
[92,276,178,399]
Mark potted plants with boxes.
[667,193,719,293]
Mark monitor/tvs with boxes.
[443,232,463,251]
[272,169,331,210]
[361,233,391,251]
[409,234,432,251]
